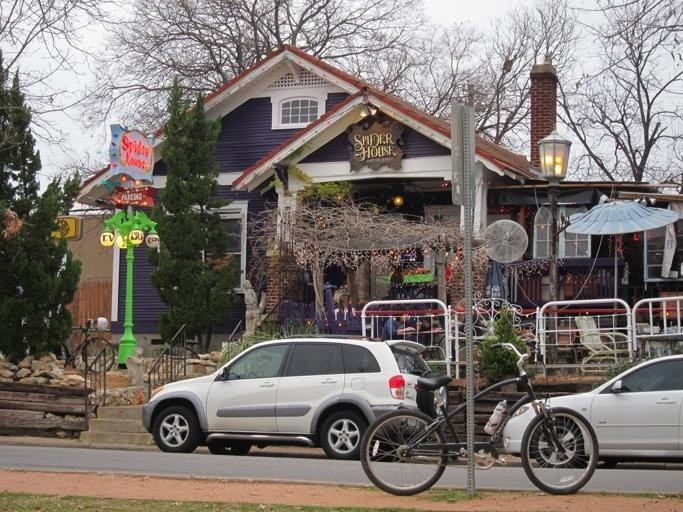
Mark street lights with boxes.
[99,204,161,365]
[536,122,574,379]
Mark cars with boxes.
[500,353,683,468]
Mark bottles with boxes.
[483,398,507,436]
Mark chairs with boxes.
[574,315,670,373]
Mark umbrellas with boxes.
[558,199,678,308]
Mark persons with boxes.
[401,314,428,345]
[379,314,415,372]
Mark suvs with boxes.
[141,330,453,461]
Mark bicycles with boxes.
[55,316,116,373]
[361,337,602,498]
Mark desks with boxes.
[400,329,445,362]
[635,333,682,359]
